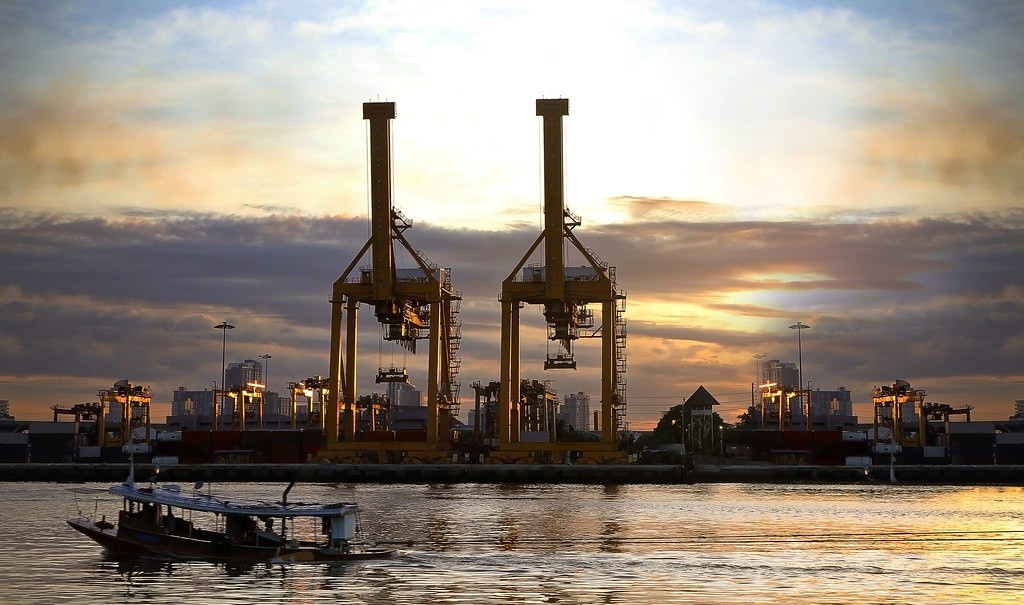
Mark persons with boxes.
[133,504,155,521]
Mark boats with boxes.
[65,449,400,564]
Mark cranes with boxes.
[323,95,467,453]
[490,95,633,458]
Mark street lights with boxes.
[788,323,811,430]
[212,380,218,432]
[214,321,236,432]
[257,353,272,390]
